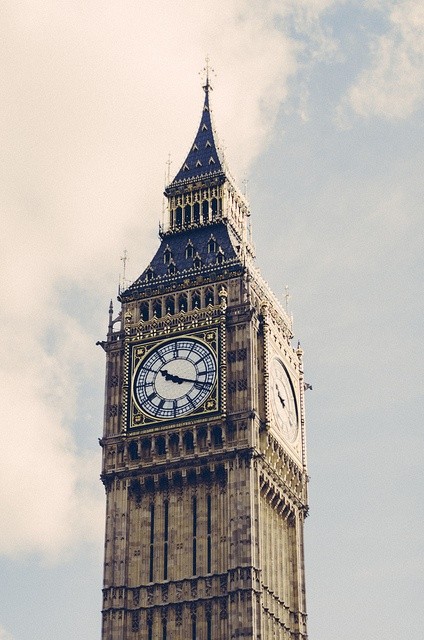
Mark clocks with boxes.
[131,336,218,421]
[268,354,299,444]
